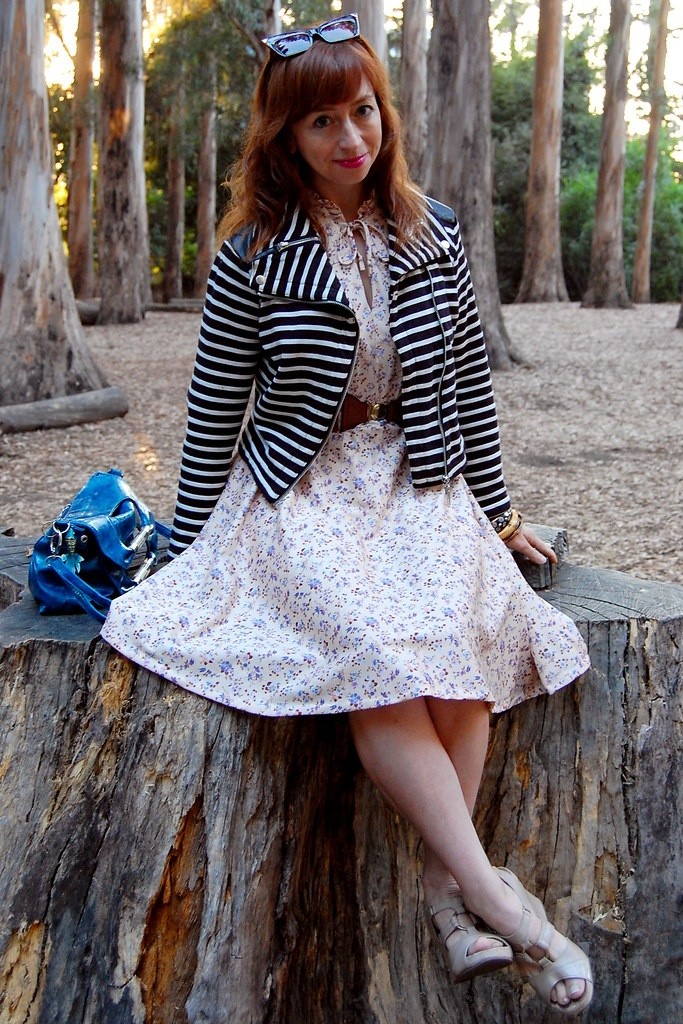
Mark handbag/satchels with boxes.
[28,469,172,624]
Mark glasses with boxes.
[261,12,360,57]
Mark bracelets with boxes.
[498,508,518,539]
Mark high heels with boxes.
[422,894,515,979]
[479,866,593,1015]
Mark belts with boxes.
[332,395,402,432]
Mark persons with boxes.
[164,23,594,1017]
